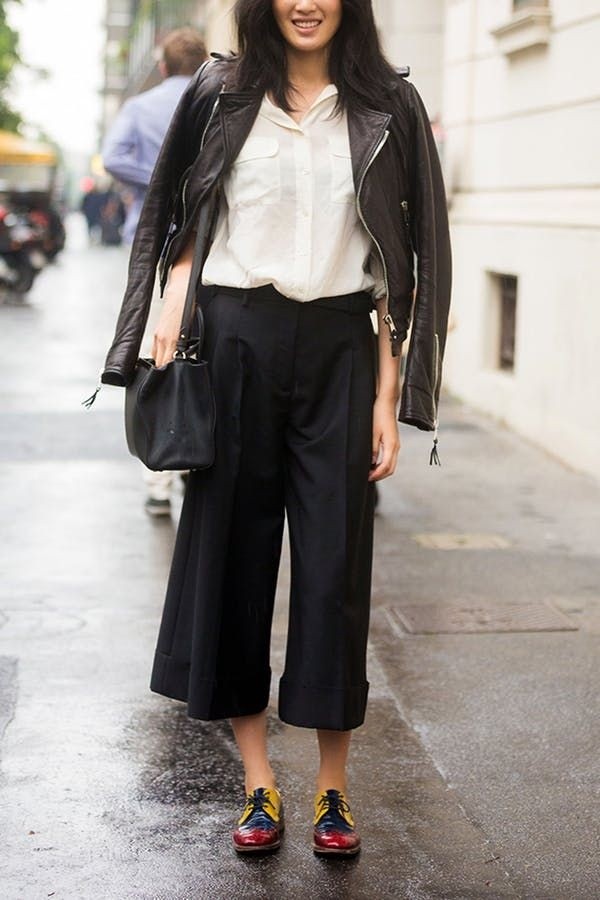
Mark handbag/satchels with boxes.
[125,353,220,471]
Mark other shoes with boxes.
[145,497,171,514]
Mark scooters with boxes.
[0,190,65,295]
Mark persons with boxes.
[103,27,209,518]
[101,0,452,861]
[81,181,126,246]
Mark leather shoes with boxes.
[311,788,362,854]
[233,785,285,852]
